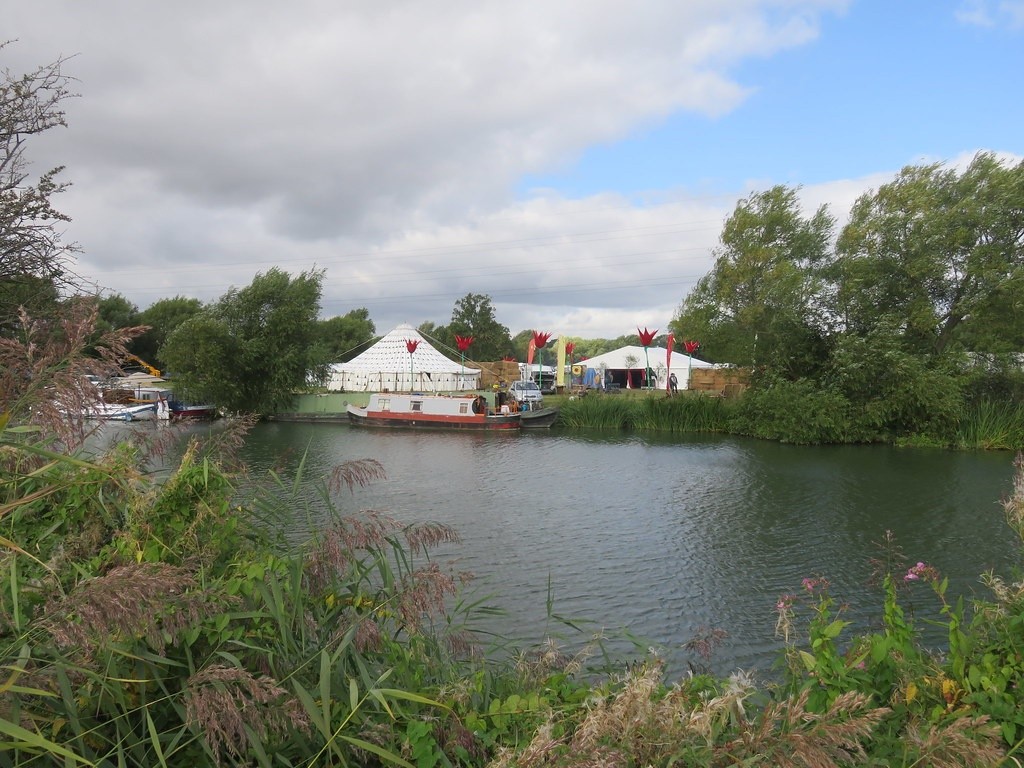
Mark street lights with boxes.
[136,381,143,401]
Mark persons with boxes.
[668,373,678,393]
[518,400,527,412]
[604,370,614,389]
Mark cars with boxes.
[510,380,544,407]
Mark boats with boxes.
[28,398,156,423]
[168,404,220,418]
[517,406,559,429]
[346,390,520,429]
